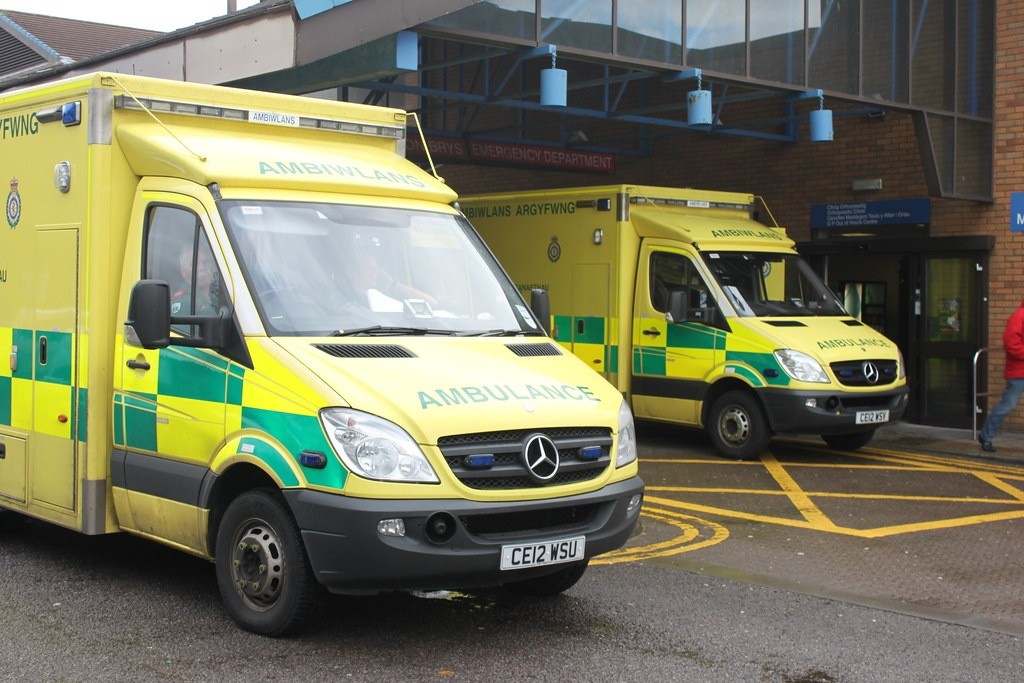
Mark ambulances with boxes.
[428,186,909,461]
[1,71,643,642]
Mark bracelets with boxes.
[424,296,433,302]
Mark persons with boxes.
[170,242,216,317]
[978,299,1024,451]
[334,219,436,309]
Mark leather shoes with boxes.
[978,436,996,452]
